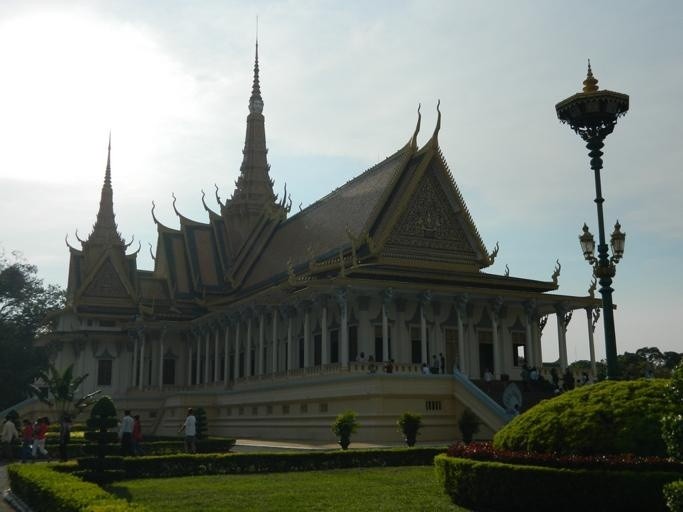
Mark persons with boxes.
[133,413,145,455]
[483,368,495,392]
[512,403,520,415]
[179,408,197,454]
[119,409,136,456]
[356,351,446,374]
[552,379,582,397]
[0,415,51,462]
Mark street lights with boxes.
[553,58,635,380]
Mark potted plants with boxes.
[457,408,483,445]
[332,409,361,450]
[396,411,424,448]
